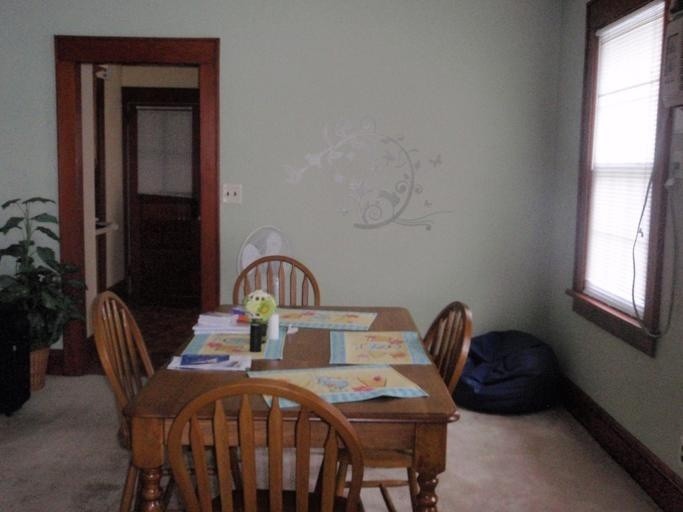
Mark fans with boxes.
[237,224,297,286]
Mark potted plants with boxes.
[0,192,88,395]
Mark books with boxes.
[193,311,251,336]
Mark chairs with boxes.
[166,378,365,512]
[313,299,473,512]
[232,255,320,308]
[90,289,244,512]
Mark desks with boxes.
[120,304,461,512]
